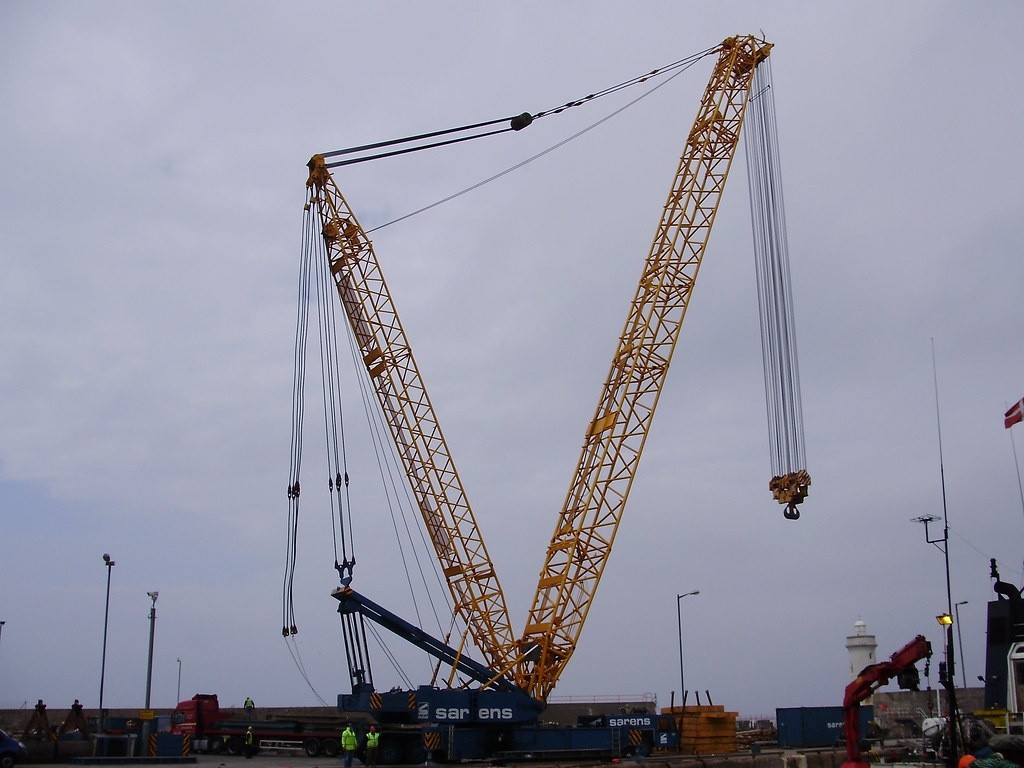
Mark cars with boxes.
[0,730,27,768]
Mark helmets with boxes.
[370,726,376,732]
[347,723,353,727]
[958,754,975,768]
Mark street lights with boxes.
[96,551,116,734]
[936,613,953,683]
[955,600,968,687]
[676,589,701,709]
[177,659,181,706]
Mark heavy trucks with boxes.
[170,693,364,758]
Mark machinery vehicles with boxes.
[285,33,812,765]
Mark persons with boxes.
[363,725,381,768]
[245,726,254,759]
[175,711,185,723]
[341,723,357,768]
[243,697,255,721]
[50,721,65,733]
